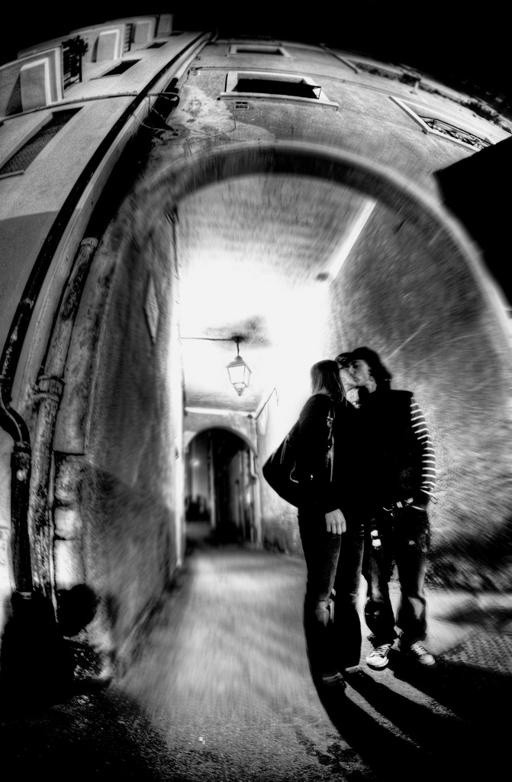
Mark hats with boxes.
[335,347,379,365]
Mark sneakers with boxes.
[411,642,435,666]
[365,643,392,668]
[322,665,363,685]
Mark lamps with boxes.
[227,335,252,398]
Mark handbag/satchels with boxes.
[261,422,299,508]
